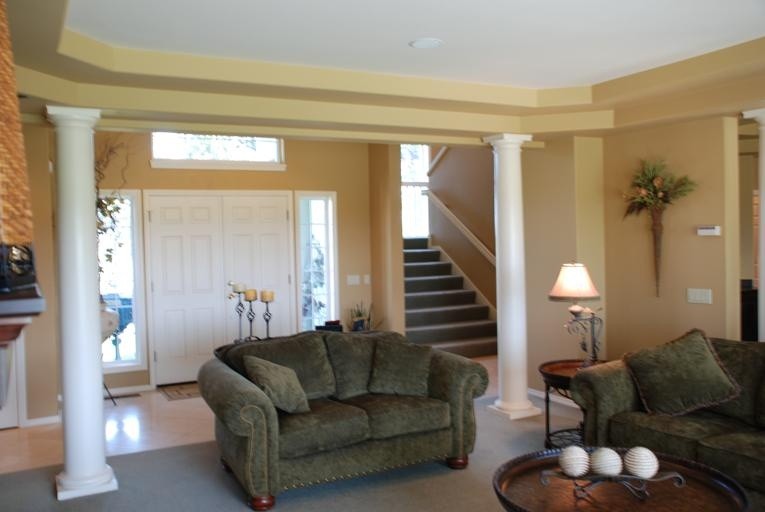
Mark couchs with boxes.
[568,329,764,501]
[196,325,491,512]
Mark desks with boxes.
[535,358,621,449]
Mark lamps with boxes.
[548,261,606,372]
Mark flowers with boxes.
[617,154,698,221]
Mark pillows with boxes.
[366,333,435,399]
[322,330,381,401]
[620,327,741,423]
[222,328,338,403]
[241,353,312,417]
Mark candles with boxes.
[242,288,257,301]
[232,282,248,294]
[258,289,273,303]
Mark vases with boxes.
[648,207,666,298]
